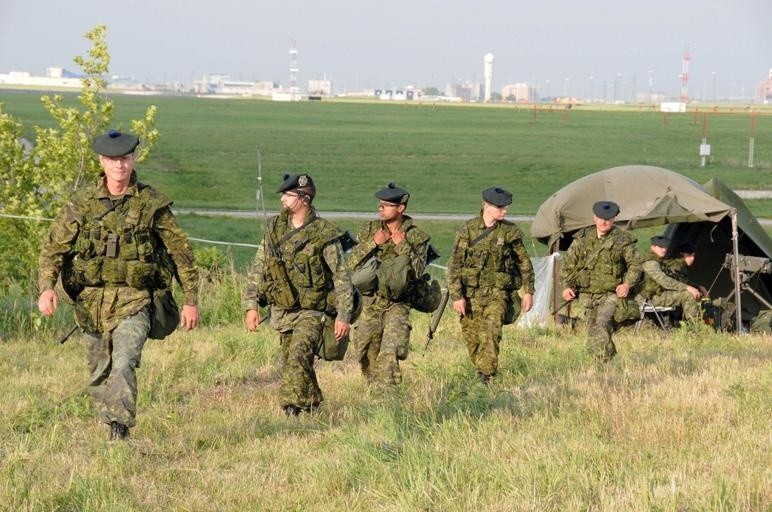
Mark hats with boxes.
[92,127,141,158]
[649,235,673,248]
[373,182,411,205]
[592,201,621,220]
[274,172,315,193]
[481,186,513,208]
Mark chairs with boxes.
[633,298,676,335]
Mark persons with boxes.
[35,127,200,442]
[442,185,536,383]
[240,173,357,416]
[660,244,697,279]
[346,180,430,394]
[636,235,708,330]
[558,200,645,359]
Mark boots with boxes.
[109,422,129,442]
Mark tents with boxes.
[528,163,772,354]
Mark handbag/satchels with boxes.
[148,282,183,341]
[502,290,523,326]
[349,255,381,296]
[314,314,351,362]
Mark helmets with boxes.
[412,279,444,314]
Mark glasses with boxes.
[279,192,302,198]
[377,200,400,208]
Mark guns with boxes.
[649,298,666,332]
[632,298,647,333]
[250,231,358,332]
[422,289,449,357]
[551,293,578,315]
[60,324,78,344]
